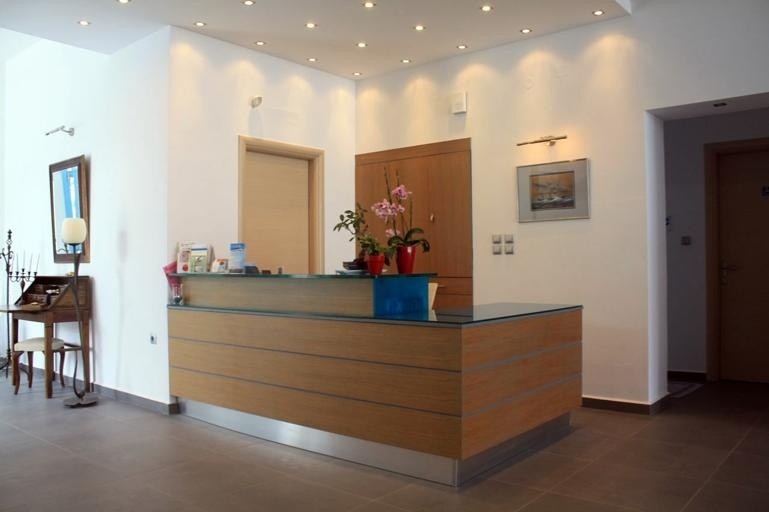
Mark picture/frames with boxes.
[514,156,592,222]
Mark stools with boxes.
[11,337,69,395]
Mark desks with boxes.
[6,273,93,398]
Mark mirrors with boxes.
[46,152,90,266]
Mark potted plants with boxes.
[330,202,389,274]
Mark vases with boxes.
[396,243,416,272]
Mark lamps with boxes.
[56,215,97,406]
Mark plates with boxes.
[336,269,388,275]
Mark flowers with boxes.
[372,169,434,252]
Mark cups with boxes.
[171,283,184,306]
